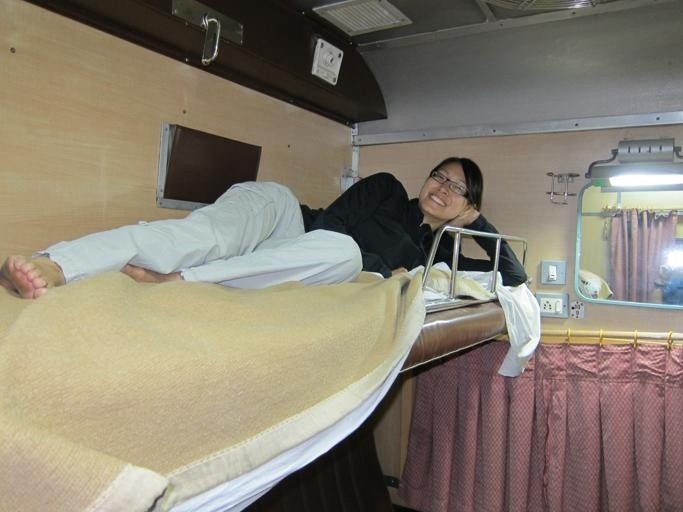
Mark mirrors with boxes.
[574,177,683,309]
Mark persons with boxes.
[0,156,527,301]
[651,238,683,305]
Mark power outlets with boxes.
[532,292,570,319]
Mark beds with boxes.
[1,227,529,385]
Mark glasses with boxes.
[429,168,472,200]
